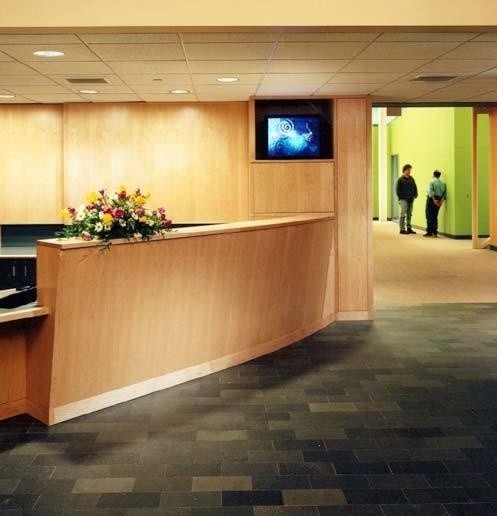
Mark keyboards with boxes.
[0,288,37,308]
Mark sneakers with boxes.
[423,232,437,237]
[400,229,416,235]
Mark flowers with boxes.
[54,184,177,256]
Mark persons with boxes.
[423,169,446,236]
[395,163,418,234]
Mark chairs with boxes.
[0,257,37,290]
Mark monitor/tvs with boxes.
[262,113,322,159]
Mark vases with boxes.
[97,224,131,238]
